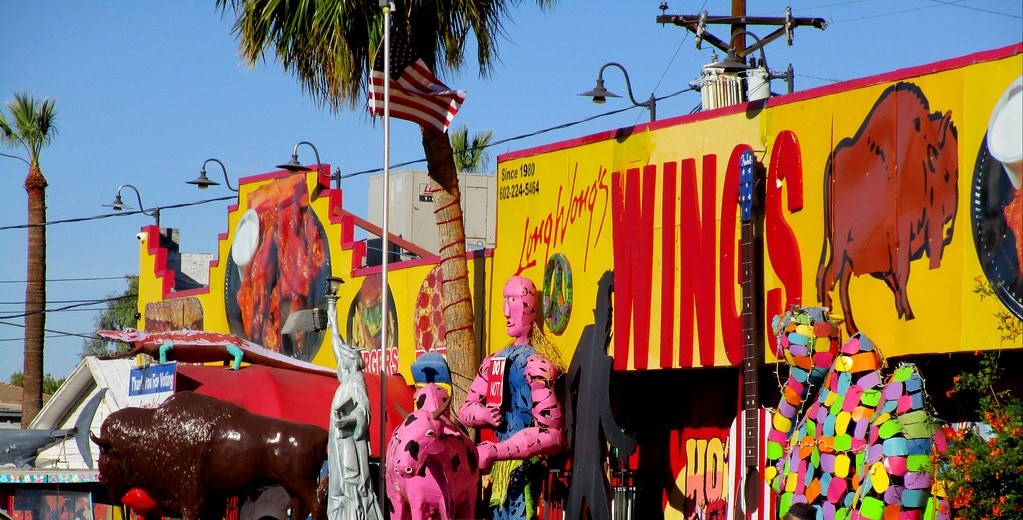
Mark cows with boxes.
[88,390,330,520]
[384,395,480,520]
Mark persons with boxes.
[327,309,385,519]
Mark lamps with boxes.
[705,31,794,95]
[185,159,239,193]
[274,141,340,189]
[577,61,651,113]
[102,181,159,221]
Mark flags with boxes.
[369,27,467,137]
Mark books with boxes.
[335,397,357,438]
